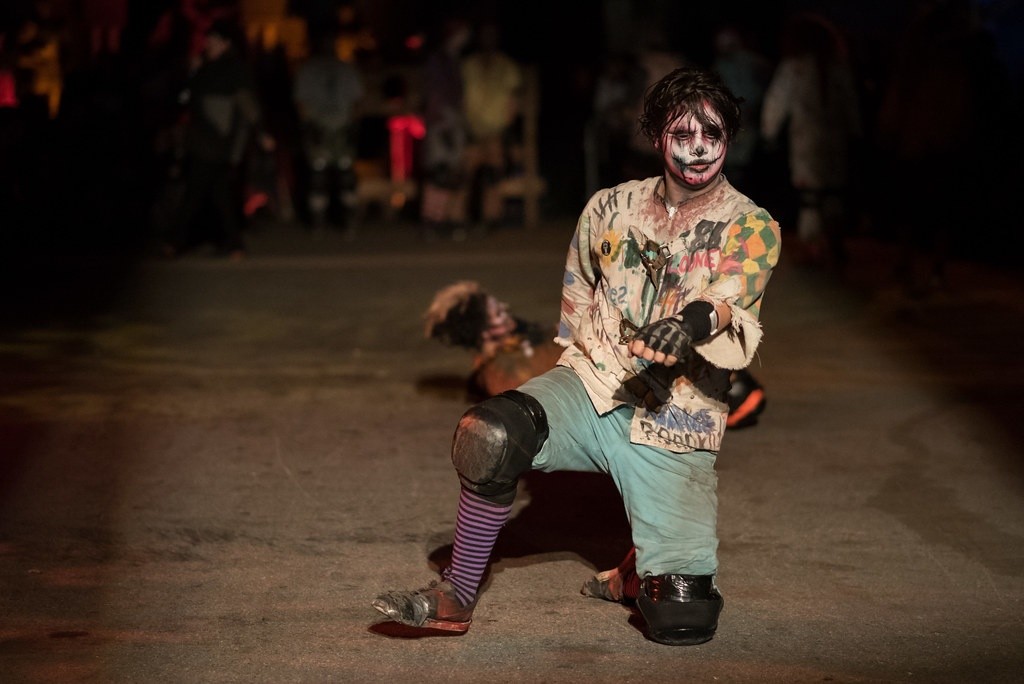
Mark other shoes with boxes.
[581,549,639,602]
[372,578,473,631]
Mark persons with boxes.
[424,282,768,429]
[369,64,785,646]
[0,0,1006,301]
